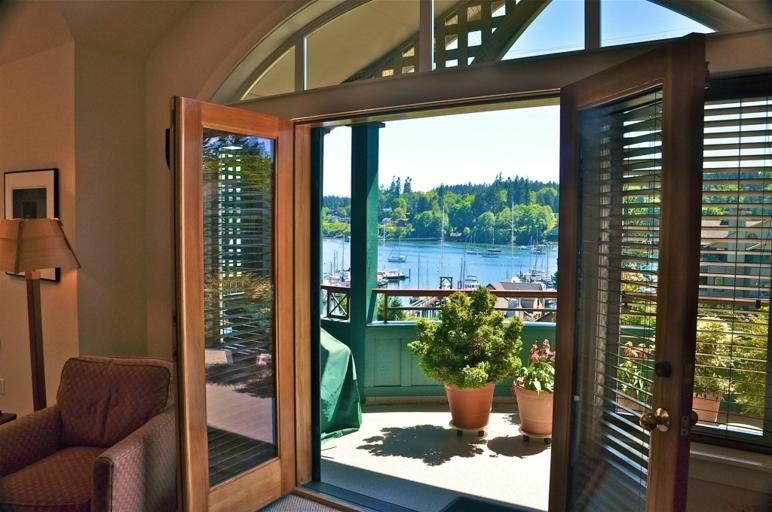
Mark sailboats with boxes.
[466,227,502,257]
[499,256,554,283]
[518,227,547,255]
[323,223,406,289]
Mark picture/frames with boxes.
[3,167,58,284]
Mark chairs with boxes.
[1,352,178,512]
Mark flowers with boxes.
[517,339,652,404]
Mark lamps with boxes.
[0,216,82,412]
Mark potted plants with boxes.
[407,284,524,433]
[685,314,733,423]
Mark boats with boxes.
[464,275,480,288]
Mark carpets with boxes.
[441,496,527,512]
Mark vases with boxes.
[512,380,645,439]
